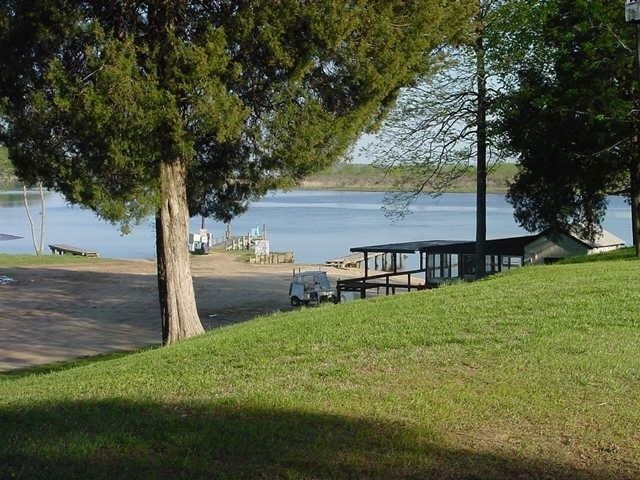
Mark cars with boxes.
[290,270,338,307]
